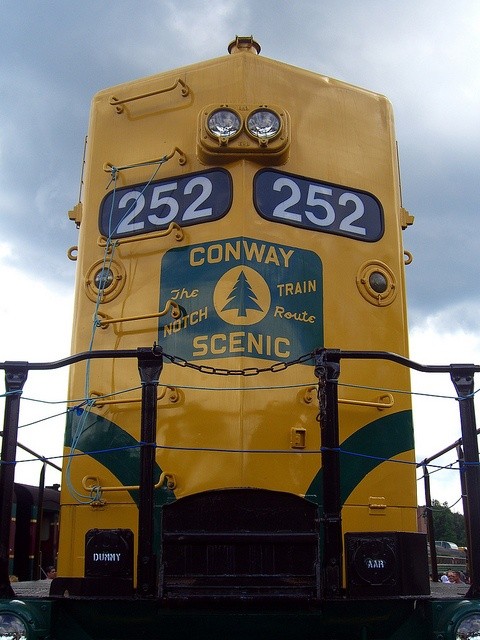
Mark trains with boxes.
[1,34,480,640]
[9,482,61,583]
[435,541,458,551]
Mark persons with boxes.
[46,566,56,579]
[460,569,466,582]
[452,571,467,584]
[445,571,455,583]
[441,572,448,583]
